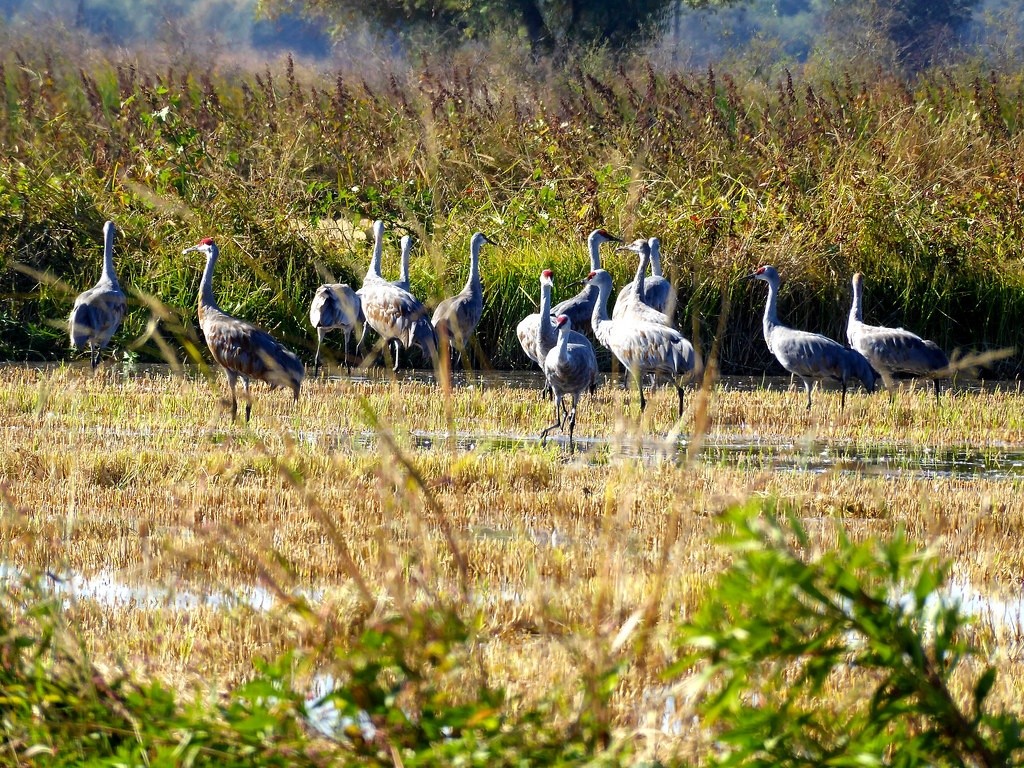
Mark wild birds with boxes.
[66,219,944,459]
